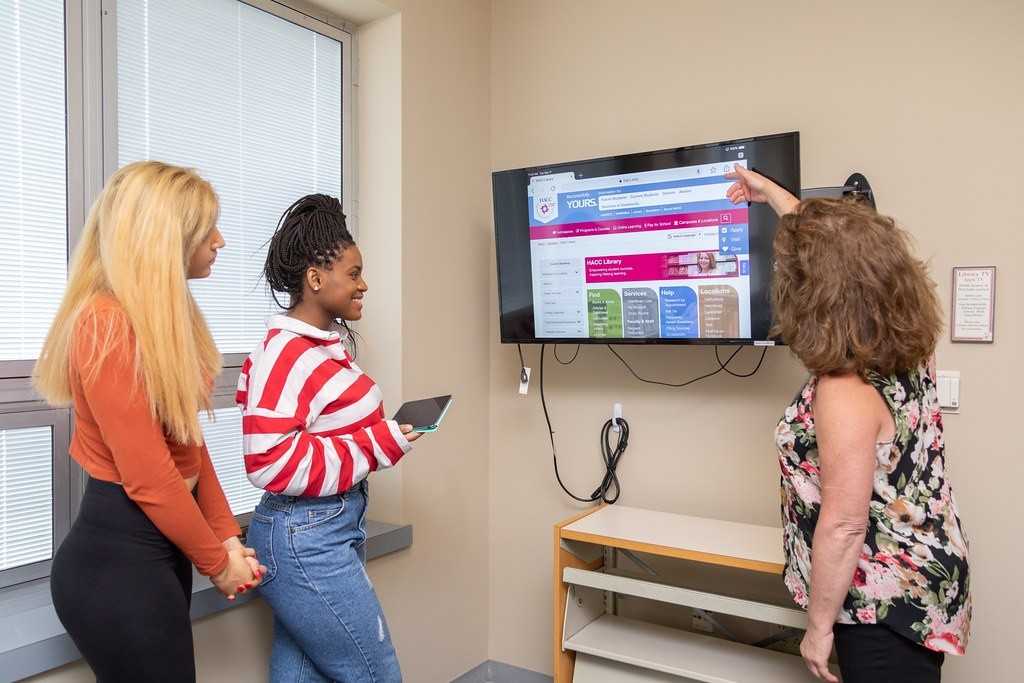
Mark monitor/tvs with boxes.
[493,130,803,347]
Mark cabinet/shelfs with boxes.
[554,503,843,683]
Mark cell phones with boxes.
[390,395,453,430]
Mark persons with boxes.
[694,252,722,276]
[29,159,267,683]
[721,162,972,683]
[236,194,422,683]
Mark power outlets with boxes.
[769,623,806,648]
[692,608,714,632]
